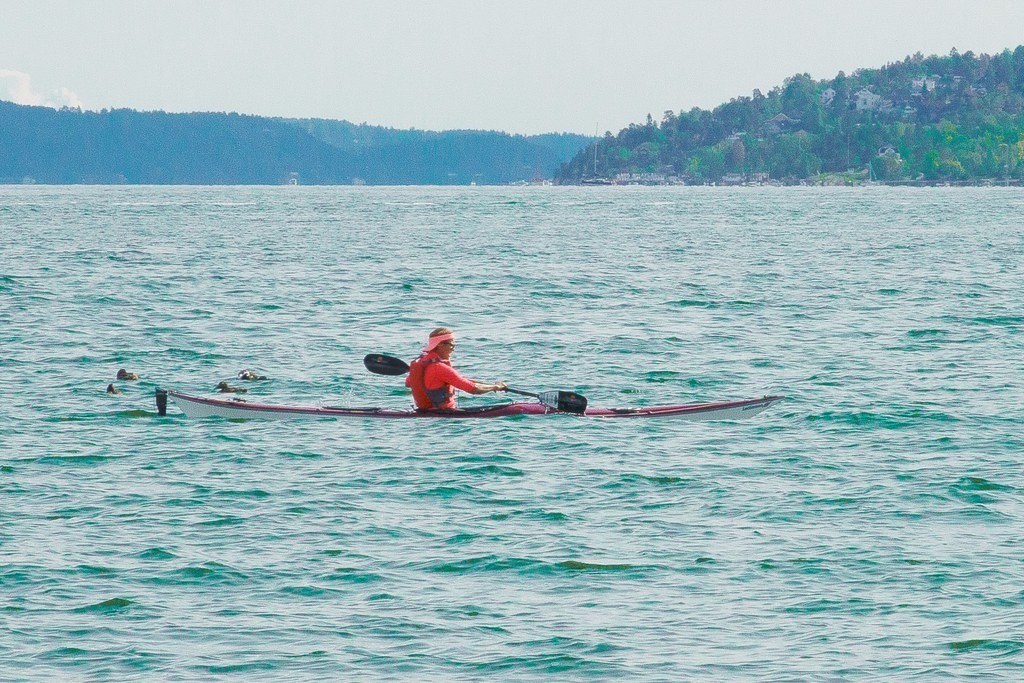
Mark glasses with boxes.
[444,342,455,349]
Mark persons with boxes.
[406,327,506,410]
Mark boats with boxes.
[171,392,785,422]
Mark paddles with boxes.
[362,353,590,417]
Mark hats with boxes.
[422,332,454,352]
